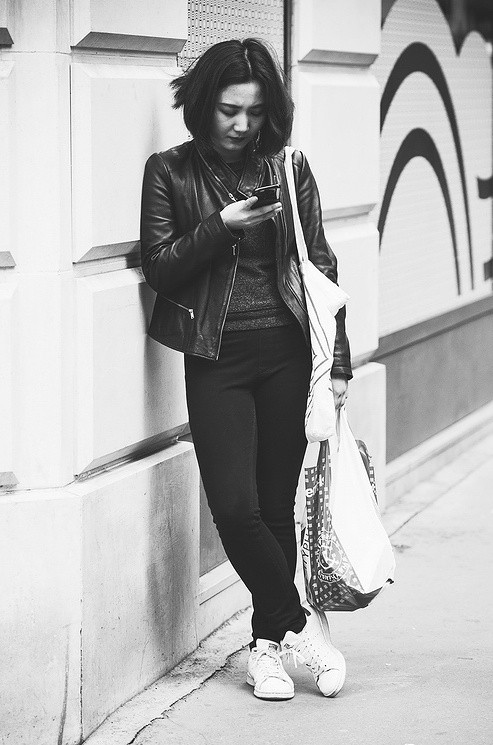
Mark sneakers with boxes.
[247,639,294,700]
[280,603,346,697]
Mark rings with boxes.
[339,394,341,397]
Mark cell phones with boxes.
[250,184,282,210]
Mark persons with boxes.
[139,37,352,699]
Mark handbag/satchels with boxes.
[283,145,350,443]
[294,400,396,614]
